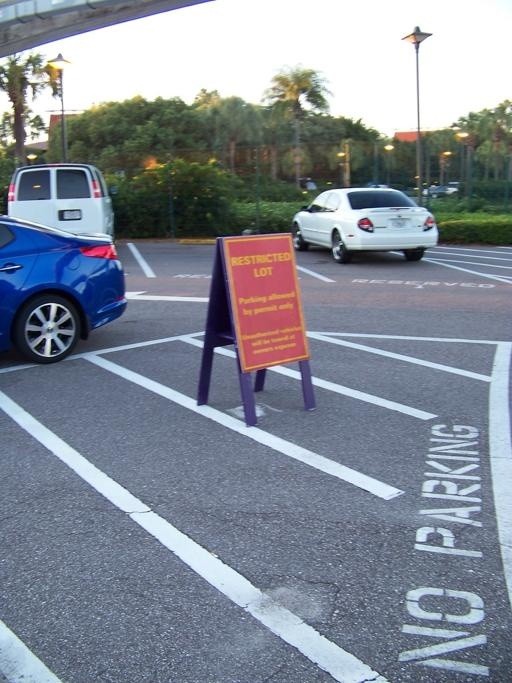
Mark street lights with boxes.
[401,26,433,206]
[47,53,71,164]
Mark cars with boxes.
[299,177,459,199]
[0,212,128,363]
[291,187,439,262]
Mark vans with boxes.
[7,163,115,244]
